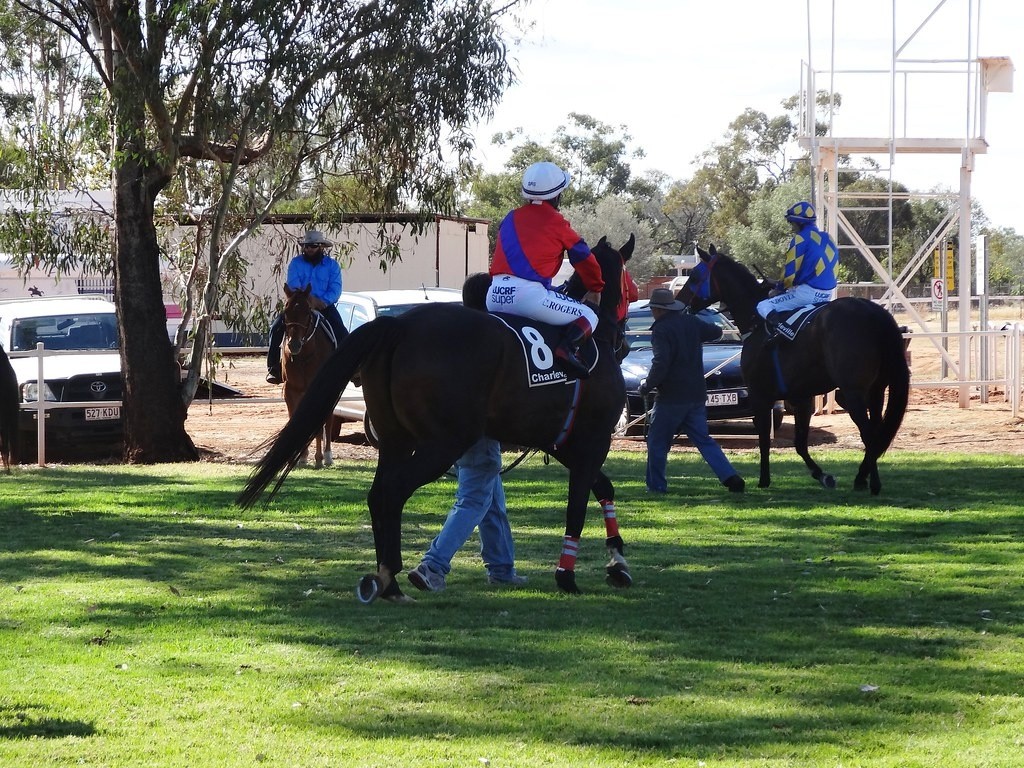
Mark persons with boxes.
[482,159,607,378]
[264,231,364,388]
[754,201,838,343]
[406,273,529,590]
[637,288,746,494]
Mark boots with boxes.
[556,316,590,377]
[766,309,785,343]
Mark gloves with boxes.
[639,384,651,396]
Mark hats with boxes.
[639,288,685,311]
[299,231,333,248]
[522,161,571,200]
[787,202,816,221]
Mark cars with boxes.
[611,300,784,436]
[0,291,134,457]
[328,284,465,450]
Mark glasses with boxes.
[302,243,324,249]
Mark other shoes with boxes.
[729,476,745,494]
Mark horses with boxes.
[240,232,636,604]
[281,283,338,469]
[675,242,911,495]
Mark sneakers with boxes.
[408,562,446,591]
[490,573,528,587]
[267,373,281,383]
